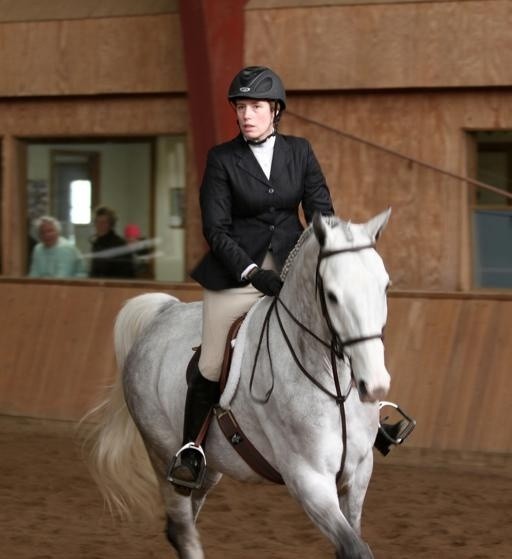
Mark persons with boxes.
[88,207,136,278]
[171,65,406,497]
[28,216,91,280]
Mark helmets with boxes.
[226,65,289,112]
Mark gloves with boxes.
[247,266,285,297]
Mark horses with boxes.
[73,206,393,559]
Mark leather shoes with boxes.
[373,419,408,457]
[169,442,203,482]
